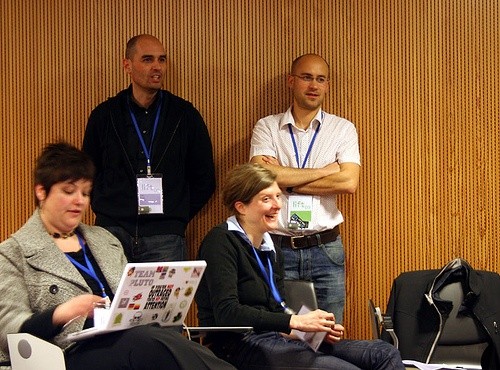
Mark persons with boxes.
[247,53,362,325]
[0,141,237,369]
[194,161,405,369]
[80,33,217,262]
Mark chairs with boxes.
[392,270,500,364]
[282,281,326,315]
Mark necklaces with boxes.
[48,230,75,238]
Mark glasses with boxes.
[293,74,326,83]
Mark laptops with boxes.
[67,260,207,341]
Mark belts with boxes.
[270,225,340,250]
